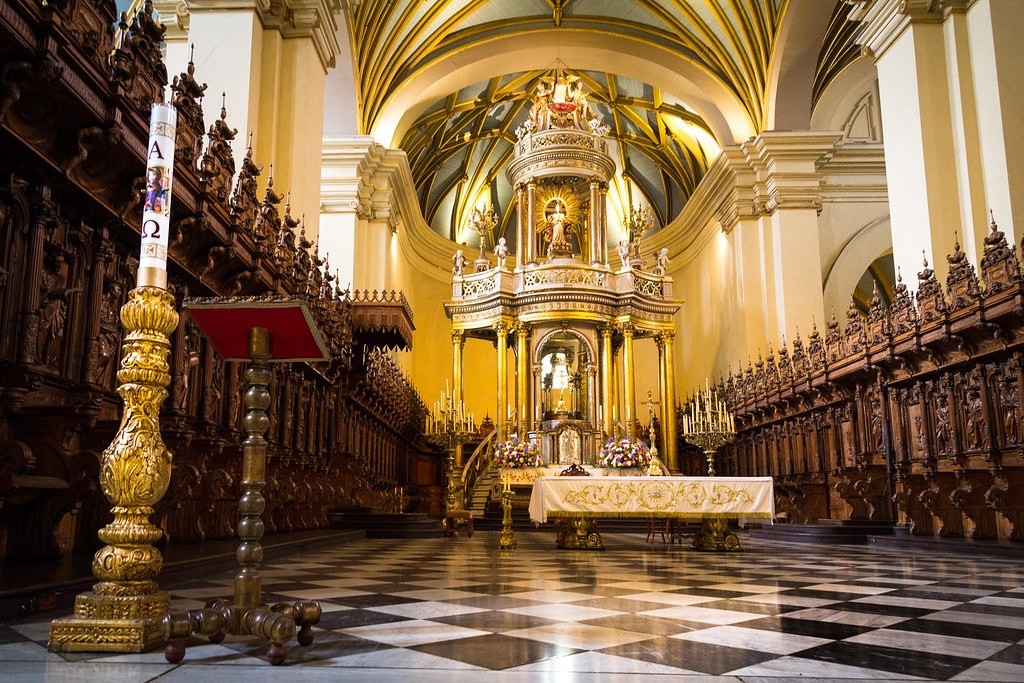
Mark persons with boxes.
[658,248,672,276]
[547,203,565,242]
[619,240,631,266]
[452,250,469,276]
[529,81,598,124]
[494,237,510,267]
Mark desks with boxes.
[528,476,775,551]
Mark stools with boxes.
[446,510,473,538]
[776,512,788,523]
[646,517,701,544]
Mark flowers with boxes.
[492,437,547,467]
[594,437,651,470]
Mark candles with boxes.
[508,404,511,419]
[628,405,631,418]
[426,379,474,433]
[508,475,510,490]
[504,476,506,490]
[536,405,538,420]
[682,378,734,436]
[600,405,602,420]
[613,405,616,420]
[137,102,177,289]
[523,405,525,419]
